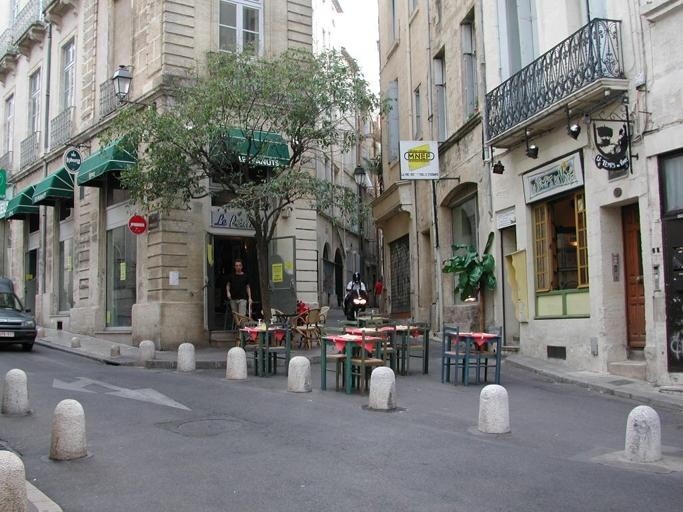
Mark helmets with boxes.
[353,272,361,281]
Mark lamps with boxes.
[489,143,506,176]
[110,64,156,119]
[564,102,581,140]
[523,125,539,160]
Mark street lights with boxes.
[352,162,370,284]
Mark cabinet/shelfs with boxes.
[555,226,579,273]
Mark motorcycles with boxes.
[342,287,370,322]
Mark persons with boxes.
[373,279,384,307]
[344,272,368,309]
[225,259,254,338]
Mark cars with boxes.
[0,275,38,350]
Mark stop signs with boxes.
[128,215,147,237]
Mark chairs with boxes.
[229,301,505,396]
